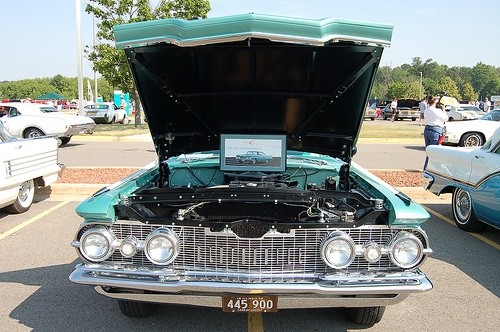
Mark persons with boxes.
[390,97,398,122]
[484,97,491,112]
[419,98,427,119]
[24,97,31,103]
[119,96,131,123]
[422,96,449,147]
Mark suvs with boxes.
[363,98,421,121]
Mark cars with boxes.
[0,101,129,214]
[68,14,434,325]
[422,108,500,231]
[236,151,273,165]
[418,96,486,121]
